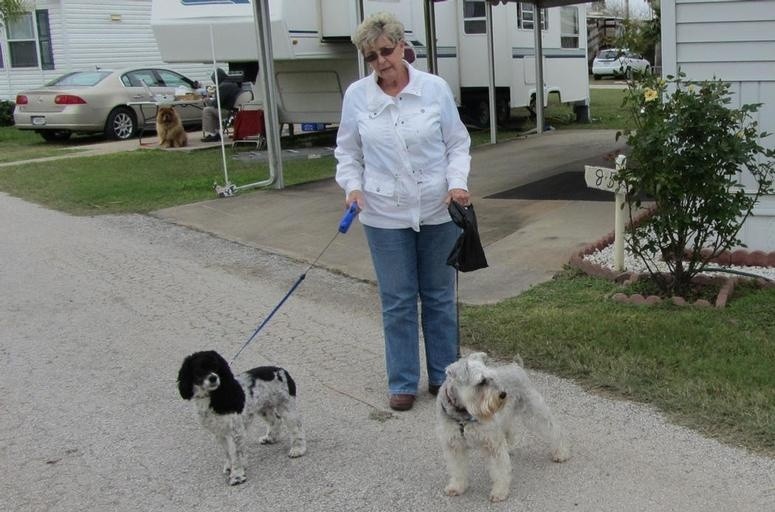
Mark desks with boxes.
[125,97,229,146]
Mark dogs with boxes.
[178,350,306,486]
[156,106,187,149]
[435,353,568,503]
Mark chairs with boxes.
[222,90,255,137]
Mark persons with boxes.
[201,68,238,142]
[334,11,472,411]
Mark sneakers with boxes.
[429,381,442,395]
[390,390,414,412]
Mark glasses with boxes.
[364,44,397,63]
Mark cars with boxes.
[13,66,209,140]
[592,48,651,79]
